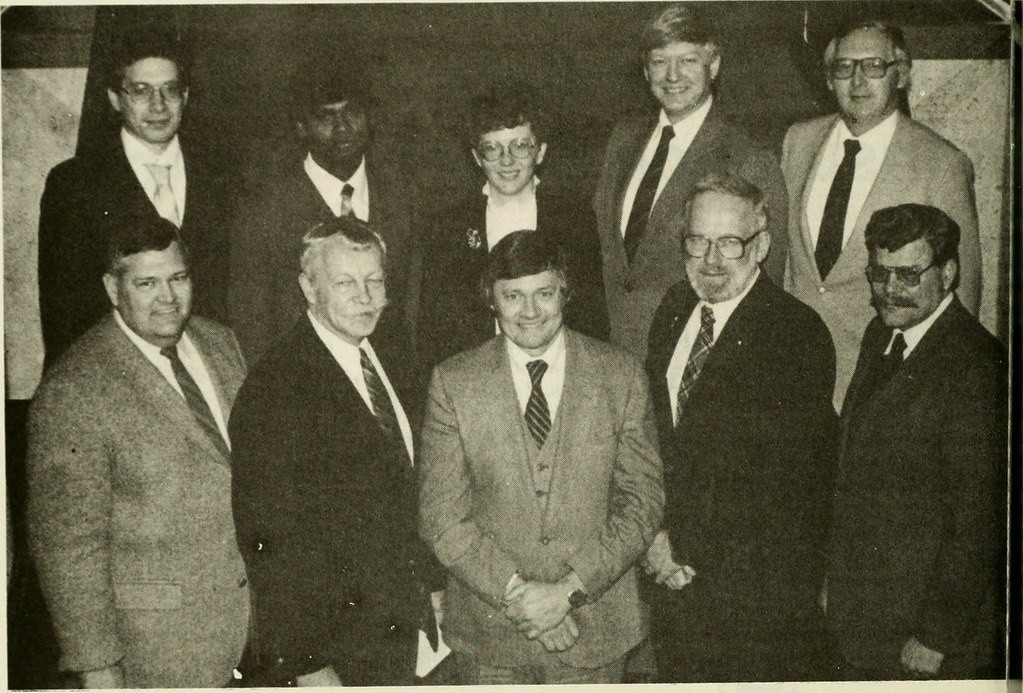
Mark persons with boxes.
[227,58,418,391]
[591,5,789,366]
[227,215,449,687]
[412,81,611,380]
[23,215,251,688]
[816,204,1009,680]
[781,12,983,415]
[36,39,228,358]
[418,230,665,685]
[635,171,840,683]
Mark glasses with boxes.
[478,137,544,165]
[111,79,188,102]
[865,264,948,285]
[679,230,762,260]
[828,56,904,81]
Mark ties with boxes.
[144,160,179,228]
[866,333,911,400]
[357,346,414,469]
[521,359,553,452]
[676,305,717,426]
[158,345,232,466]
[339,183,358,220]
[811,136,862,277]
[623,124,676,264]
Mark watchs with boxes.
[559,575,586,608]
